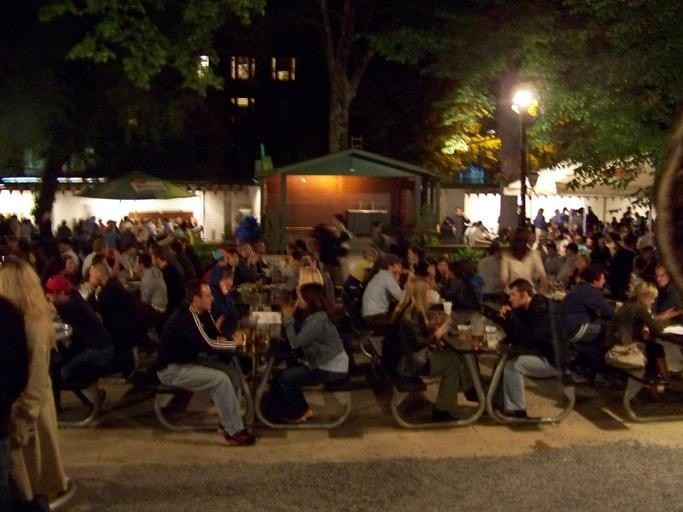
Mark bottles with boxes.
[118,271,127,289]
[51,315,64,333]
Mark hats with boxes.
[47,277,73,291]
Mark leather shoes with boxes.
[432,410,460,420]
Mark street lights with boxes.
[509,86,541,229]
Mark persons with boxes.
[0,203,682,450]
[1,243,75,510]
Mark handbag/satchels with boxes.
[605,344,646,368]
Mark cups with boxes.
[615,302,623,313]
[234,257,284,348]
[442,301,498,350]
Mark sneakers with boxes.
[218,426,255,444]
